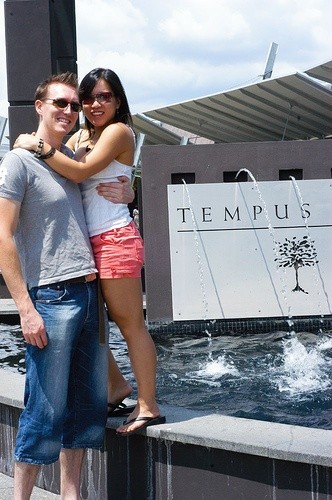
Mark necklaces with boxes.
[84,145,92,152]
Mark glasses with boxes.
[40,97,82,112]
[82,92,115,105]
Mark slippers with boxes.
[107,400,135,417]
[114,415,166,437]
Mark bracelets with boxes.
[34,138,43,158]
[37,144,56,159]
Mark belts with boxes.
[66,273,99,283]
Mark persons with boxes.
[13,68,166,436]
[0,74,134,500]
